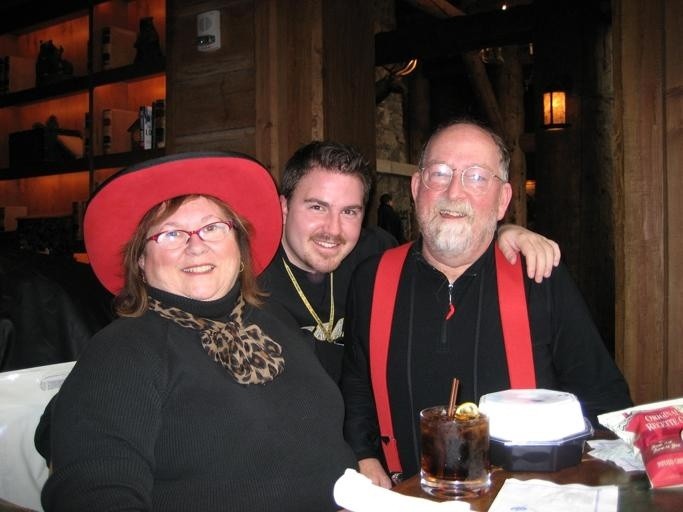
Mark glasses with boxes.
[418,164,504,196]
[144,219,234,251]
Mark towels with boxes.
[332,467,475,512]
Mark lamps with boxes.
[542,84,571,133]
[526,178,537,199]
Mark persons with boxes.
[31,186,361,512]
[375,186,410,251]
[245,141,561,382]
[338,117,639,490]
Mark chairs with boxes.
[0,361,76,512]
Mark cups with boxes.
[418,404,492,499]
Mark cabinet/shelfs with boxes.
[0,0,169,266]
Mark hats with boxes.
[83,151,283,299]
